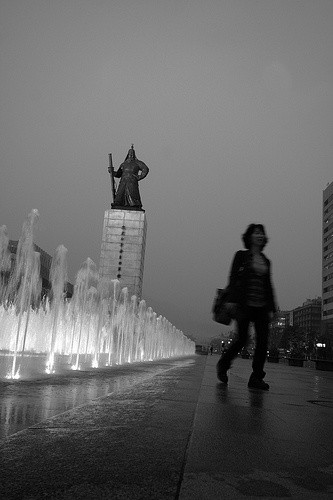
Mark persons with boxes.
[107,142,150,211]
[209,222,280,393]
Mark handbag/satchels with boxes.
[212,288,231,326]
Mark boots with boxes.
[247,369,269,389]
[216,355,231,382]
